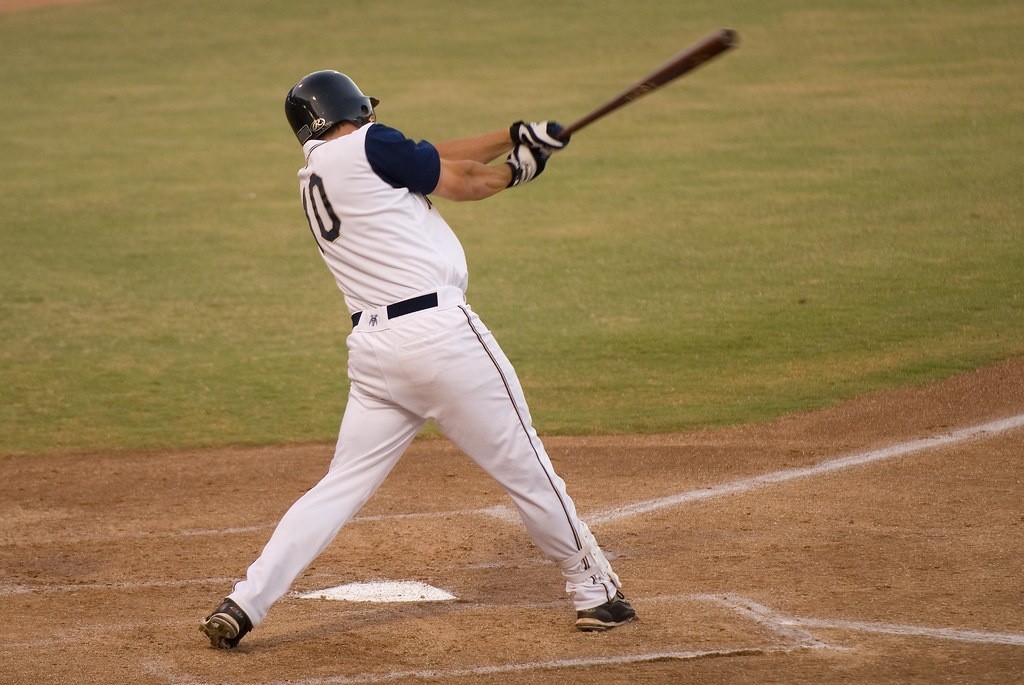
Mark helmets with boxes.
[284,69,380,147]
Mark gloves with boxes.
[503,144,554,189]
[508,119,571,150]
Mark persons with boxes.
[196,70,636,649]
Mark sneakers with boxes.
[198,597,253,649]
[575,590,635,631]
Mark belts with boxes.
[350,292,438,329]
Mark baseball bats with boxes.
[554,27,739,144]
